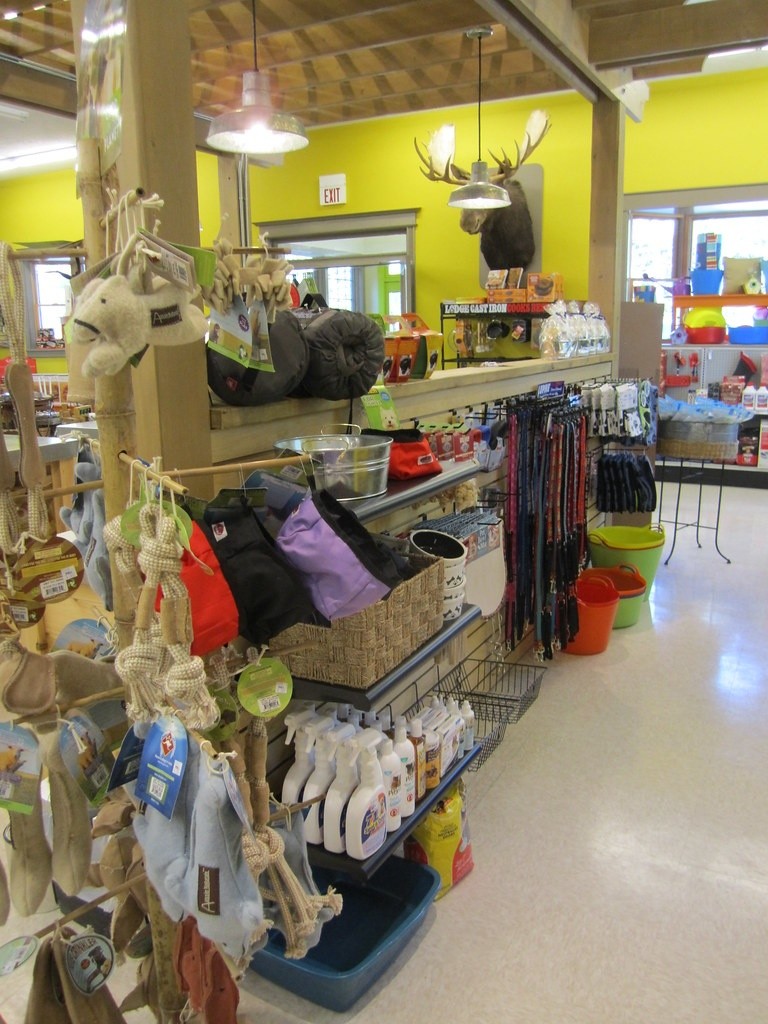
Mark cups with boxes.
[555,337,611,359]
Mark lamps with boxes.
[447,24,512,210]
[205,2,308,157]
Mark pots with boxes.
[273,423,393,501]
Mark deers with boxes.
[413,119,551,273]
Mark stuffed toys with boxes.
[69,269,210,377]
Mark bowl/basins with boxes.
[369,530,467,620]
[683,306,726,343]
[728,327,768,345]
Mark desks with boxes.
[0,419,101,539]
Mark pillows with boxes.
[719,257,763,295]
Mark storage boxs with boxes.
[727,324,768,345]
[452,270,563,305]
[249,851,443,1014]
[256,552,445,695]
[735,417,768,469]
[363,313,444,383]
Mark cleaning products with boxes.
[742,382,768,413]
[280,693,475,862]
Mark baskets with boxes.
[656,437,739,461]
[268,551,444,690]
[400,690,514,773]
[432,658,548,724]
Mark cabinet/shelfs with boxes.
[233,457,481,881]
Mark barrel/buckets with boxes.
[560,522,665,655]
[690,269,723,295]
